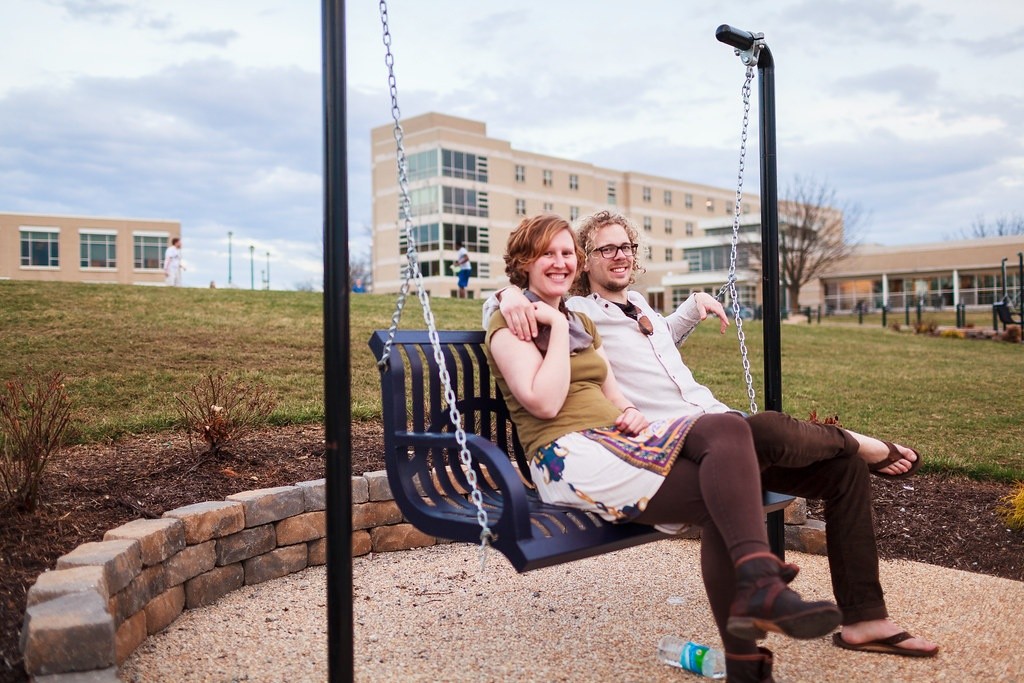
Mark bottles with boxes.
[658,635,726,679]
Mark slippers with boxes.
[834,631,939,656]
[867,441,922,481]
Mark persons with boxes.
[454,240,472,298]
[352,279,366,293]
[163,238,182,286]
[210,281,216,288]
[483,210,939,657]
[485,215,841,683]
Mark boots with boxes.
[725,646,776,683]
[727,554,845,641]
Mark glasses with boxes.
[594,242,638,258]
[622,304,653,335]
